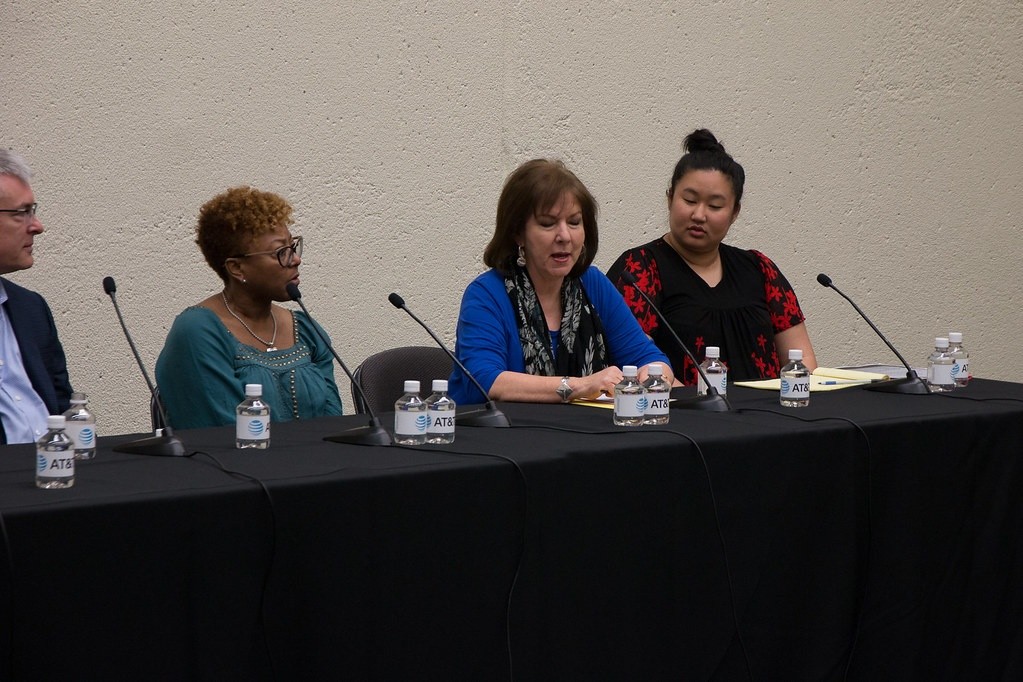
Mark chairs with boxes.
[350,347,456,414]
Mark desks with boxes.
[0,368,1023,682]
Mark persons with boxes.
[604,130,816,387]
[448,160,678,405]
[154,186,340,429]
[0,147,74,443]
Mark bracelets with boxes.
[556,376,572,403]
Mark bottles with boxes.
[394,380,427,445]
[61,392,98,459]
[612,365,647,427]
[35,414,76,489]
[947,333,970,388]
[235,383,272,450]
[424,380,457,444]
[642,365,672,425]
[697,345,728,399]
[925,338,956,393]
[780,349,810,407]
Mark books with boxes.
[734,366,890,392]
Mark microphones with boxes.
[287,282,394,446]
[620,270,730,411]
[102,276,186,456]
[816,273,930,396]
[389,293,512,429]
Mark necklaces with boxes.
[222,291,277,352]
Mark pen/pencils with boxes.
[818,379,871,384]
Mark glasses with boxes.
[0,201,38,224]
[221,236,304,267]
